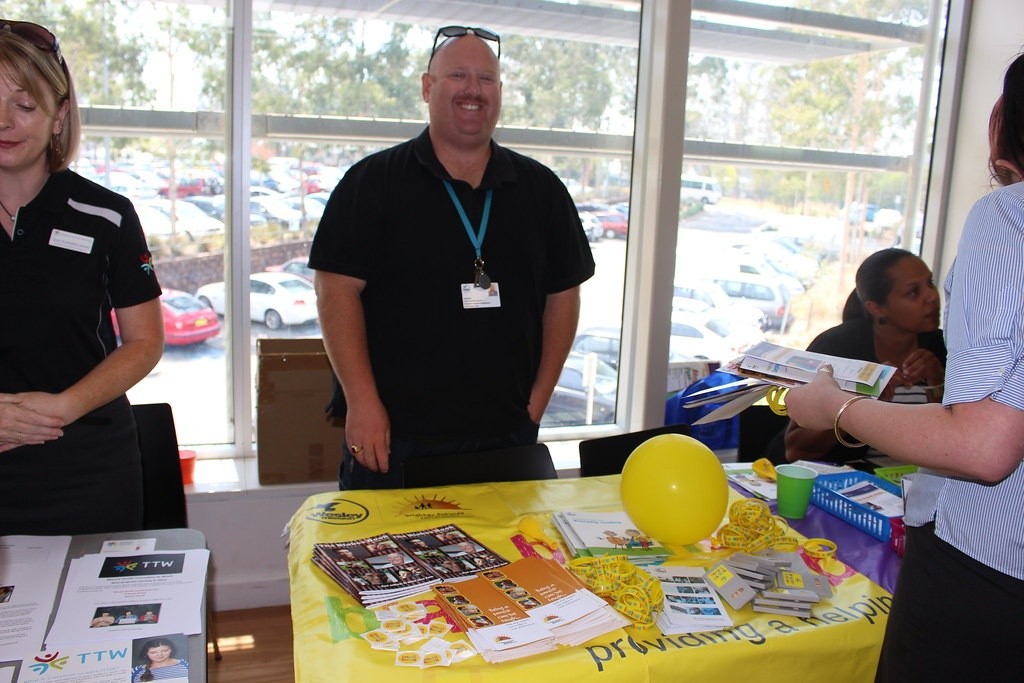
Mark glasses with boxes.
[0,19,70,99]
[427,26,501,72]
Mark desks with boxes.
[0,456,918,683]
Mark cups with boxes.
[775,464,818,519]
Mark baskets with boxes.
[811,465,919,556]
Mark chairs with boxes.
[131,402,187,528]
[734,405,790,465]
[578,423,692,476]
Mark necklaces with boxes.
[0,201,15,221]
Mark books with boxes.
[312,523,509,609]
[553,509,670,567]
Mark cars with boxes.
[195,271,319,329]
[265,256,317,286]
[538,200,881,428]
[111,287,220,351]
[68,156,354,252]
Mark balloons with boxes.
[620,433,729,546]
[753,459,776,481]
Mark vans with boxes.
[679,173,724,205]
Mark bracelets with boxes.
[922,383,943,389]
[718,499,838,557]
[568,555,662,630]
[833,395,868,447]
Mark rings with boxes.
[351,445,362,453]
[819,367,833,372]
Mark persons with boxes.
[132,639,188,683]
[0,20,165,537]
[782,53,1024,683]
[489,285,497,296]
[308,34,594,491]
[659,577,720,615]
[786,249,947,463]
[92,610,157,627]
[334,532,540,625]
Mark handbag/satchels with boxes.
[665,371,752,449]
